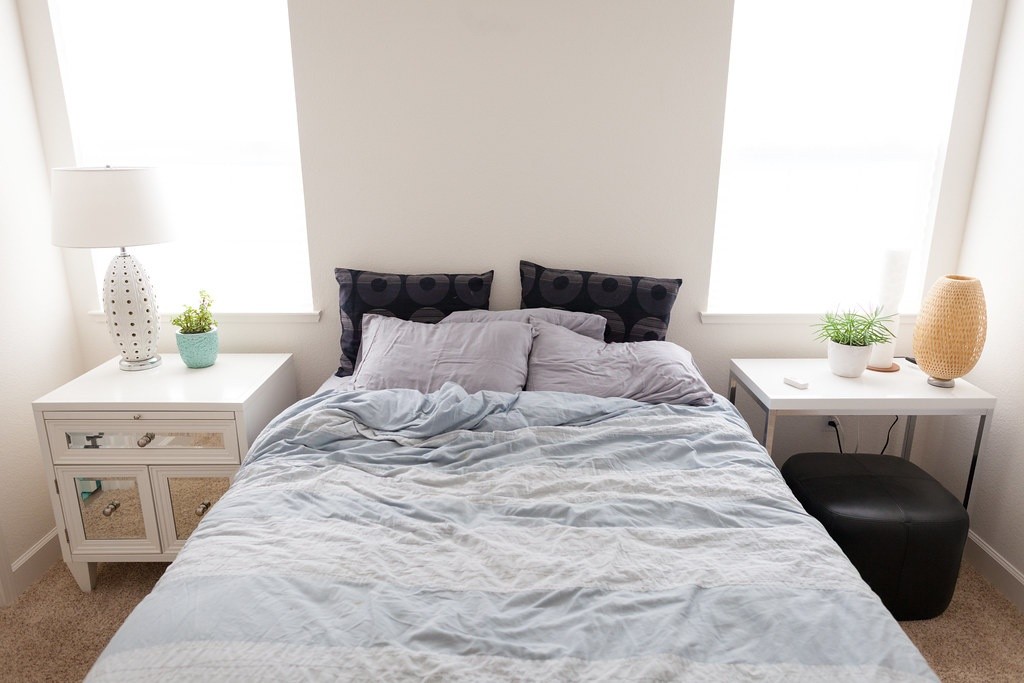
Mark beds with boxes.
[83,260,939,683]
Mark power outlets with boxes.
[821,415,842,432]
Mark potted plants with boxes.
[809,302,900,377]
[168,289,219,368]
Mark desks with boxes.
[728,357,998,510]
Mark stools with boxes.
[781,452,971,622]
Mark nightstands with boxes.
[30,352,295,592]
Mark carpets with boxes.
[0,558,175,683]
[893,553,1024,683]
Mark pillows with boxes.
[345,311,538,394]
[523,316,715,409]
[519,260,682,344]
[334,267,495,378]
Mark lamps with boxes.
[48,163,168,371]
[913,274,987,388]
